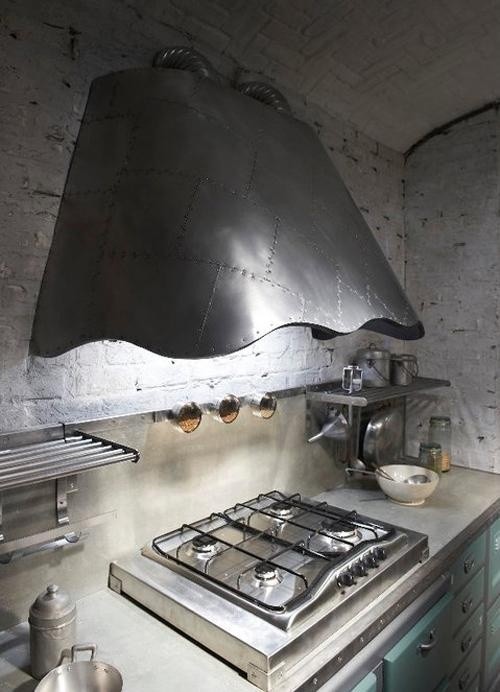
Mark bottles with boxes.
[417,415,453,476]
[28,581,78,677]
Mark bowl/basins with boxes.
[375,464,440,508]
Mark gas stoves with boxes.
[107,488,430,691]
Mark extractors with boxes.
[28,68,428,364]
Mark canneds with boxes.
[418,442,442,478]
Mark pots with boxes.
[32,642,123,692]
[362,405,406,469]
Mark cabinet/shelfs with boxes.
[350,516,498,691]
